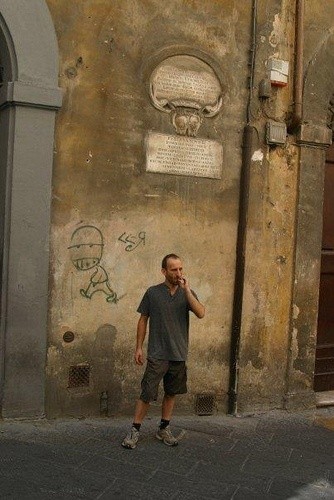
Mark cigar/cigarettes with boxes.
[179,280,183,283]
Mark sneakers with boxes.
[156,425,178,446]
[122,428,139,449]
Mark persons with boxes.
[122,252,206,449]
